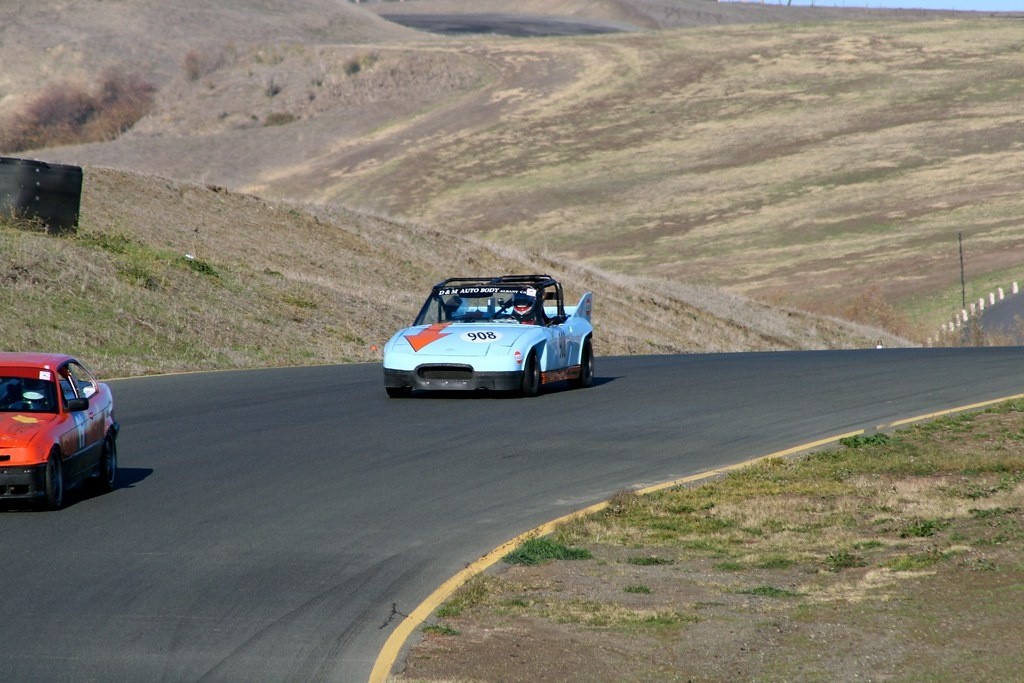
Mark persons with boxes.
[509,286,540,324]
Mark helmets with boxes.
[512,285,541,320]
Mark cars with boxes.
[381,275,594,396]
[0,351,121,511]
